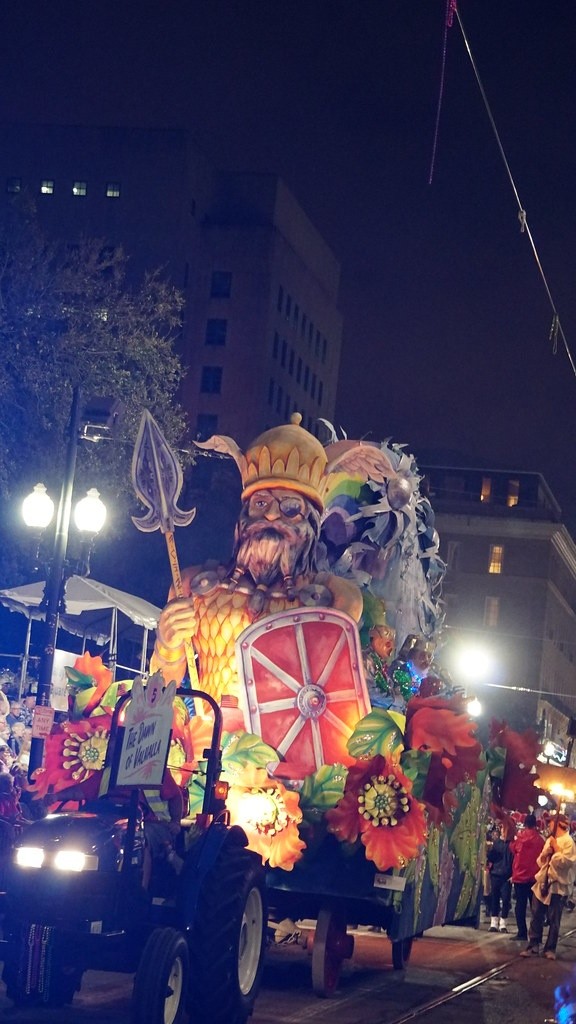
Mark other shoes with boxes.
[509,936,529,942]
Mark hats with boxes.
[535,811,576,833]
[512,812,528,822]
[367,625,395,641]
[409,637,437,653]
[544,813,569,831]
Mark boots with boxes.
[498,917,509,933]
[488,916,499,932]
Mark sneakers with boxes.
[519,944,540,957]
[544,950,557,960]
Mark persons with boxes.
[365,624,470,717]
[477,809,575,961]
[43,723,184,893]
[147,418,361,762]
[0,673,47,839]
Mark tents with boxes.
[0,574,165,701]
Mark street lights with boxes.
[19,482,111,823]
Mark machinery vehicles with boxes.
[0,688,487,1024]
